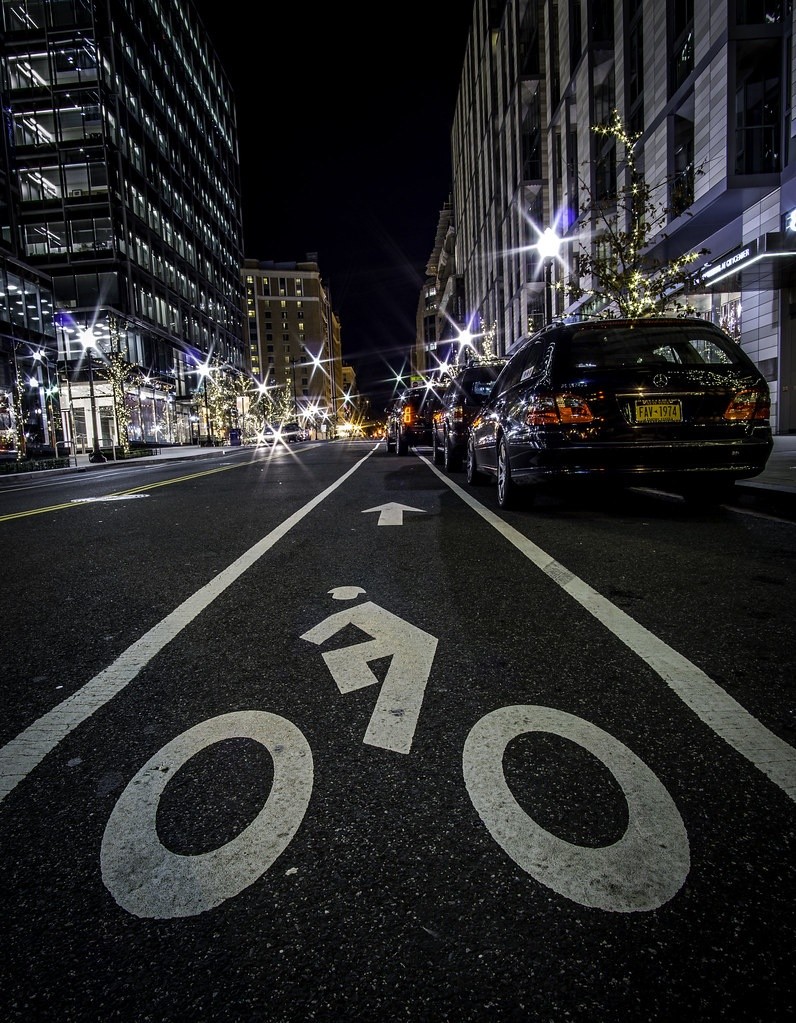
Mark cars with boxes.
[262,426,288,444]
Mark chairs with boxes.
[637,353,668,364]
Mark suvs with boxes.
[385,385,451,455]
[432,357,511,472]
[283,423,305,443]
[463,314,776,511]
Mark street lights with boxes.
[198,365,213,446]
[535,230,562,327]
[258,384,268,418]
[457,330,472,370]
[81,332,108,463]
